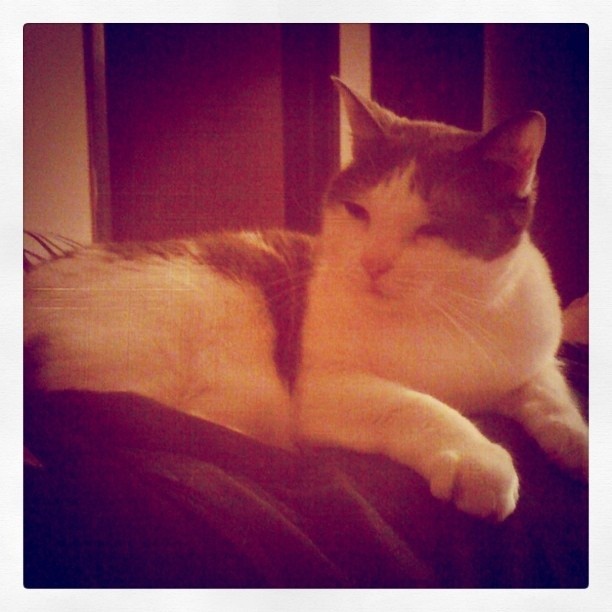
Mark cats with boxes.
[24,72,589,526]
